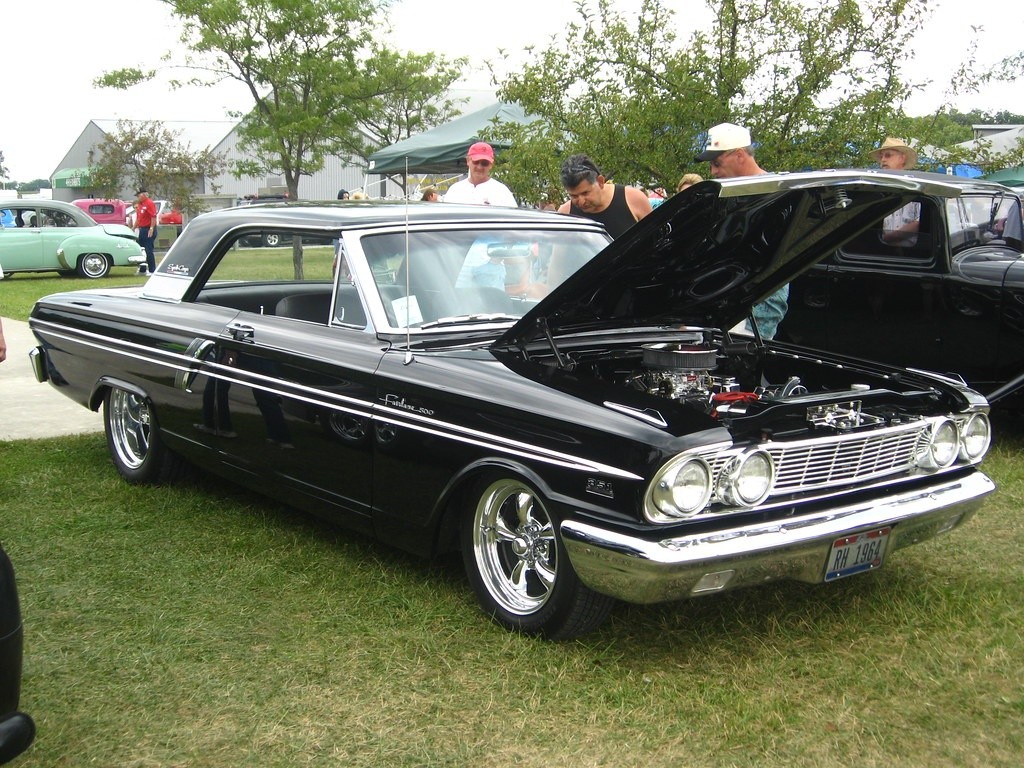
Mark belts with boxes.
[139,227,150,229]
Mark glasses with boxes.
[560,164,592,175]
[710,150,735,167]
[472,160,490,167]
[881,153,900,158]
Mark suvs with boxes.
[235,193,338,246]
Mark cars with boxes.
[1,200,149,280]
[24,169,1023,646]
[69,194,185,235]
[757,171,1024,404]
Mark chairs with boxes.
[29,216,38,227]
[443,286,514,318]
[276,293,352,326]
[14,216,25,226]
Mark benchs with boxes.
[203,285,442,327]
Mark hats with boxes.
[694,123,751,161]
[467,142,494,163]
[354,193,368,199]
[420,189,435,201]
[869,138,918,169]
[134,187,146,196]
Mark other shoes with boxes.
[137,271,146,275]
[146,272,154,276]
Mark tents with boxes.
[360,97,582,197]
[973,161,1024,187]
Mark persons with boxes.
[128,188,158,276]
[677,122,789,342]
[1003,192,1024,250]
[0,255,38,764]
[869,136,927,245]
[331,190,364,278]
[542,153,653,297]
[282,190,288,200]
[421,142,518,289]
